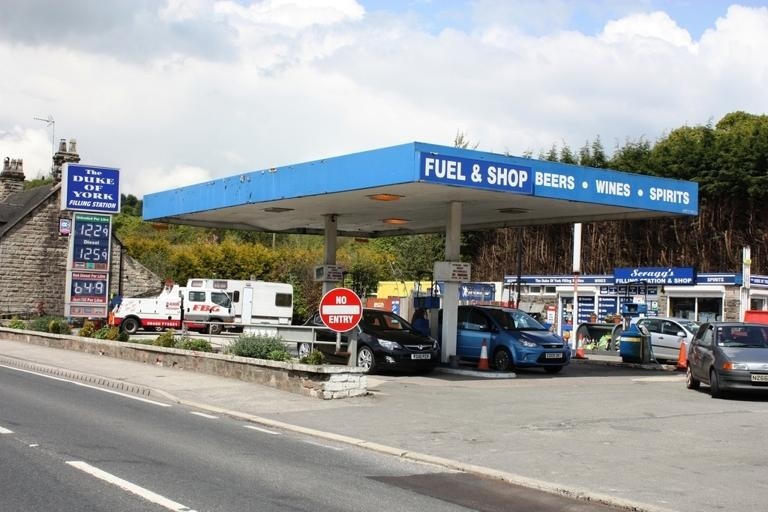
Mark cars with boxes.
[296,309,442,376]
[629,316,712,362]
[686,321,768,398]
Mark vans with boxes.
[410,305,571,374]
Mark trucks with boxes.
[108,280,236,335]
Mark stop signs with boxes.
[319,287,364,333]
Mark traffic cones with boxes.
[673,340,688,372]
[572,333,588,359]
[472,338,492,372]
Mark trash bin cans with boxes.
[637,324,651,365]
